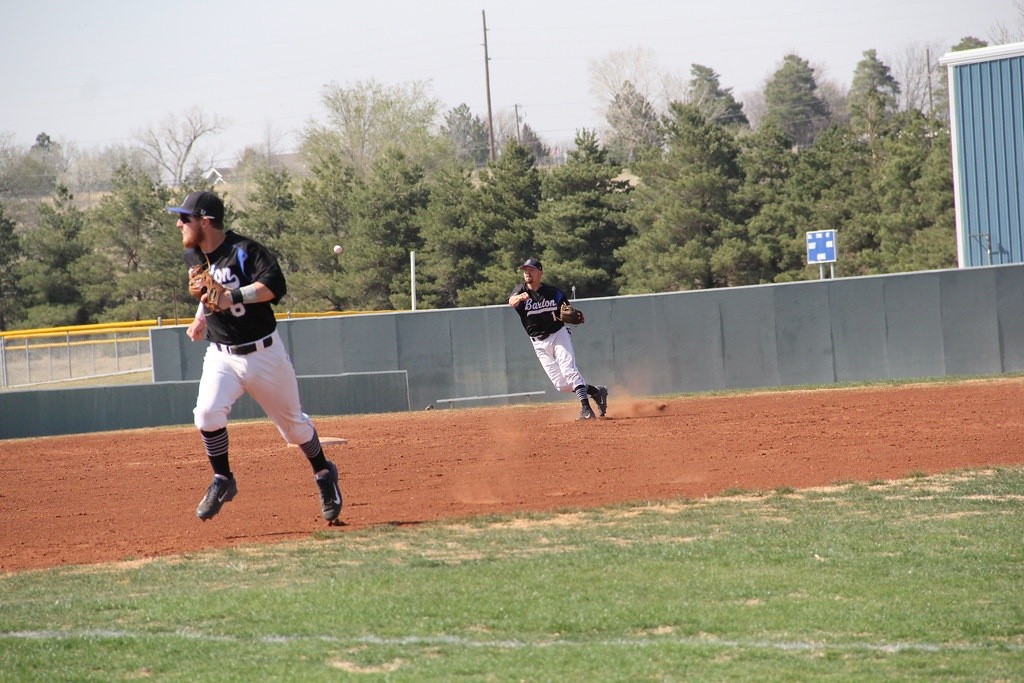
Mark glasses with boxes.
[179,213,199,224]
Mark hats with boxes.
[519,259,542,271]
[167,190,224,222]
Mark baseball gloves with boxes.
[560,304,585,325]
[187,263,226,314]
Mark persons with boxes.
[508,258,608,421]
[166,192,343,520]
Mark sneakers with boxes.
[574,407,595,421]
[591,385,608,417]
[195,473,238,521]
[312,458,343,521]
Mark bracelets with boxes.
[229,288,244,305]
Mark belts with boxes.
[532,333,550,341]
[214,336,273,355]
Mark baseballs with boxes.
[333,245,343,254]
